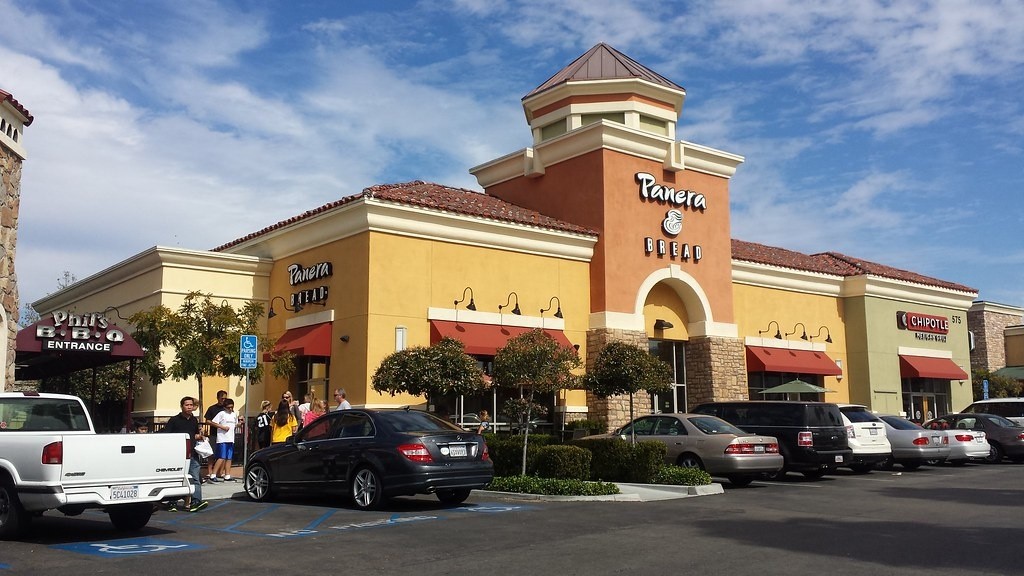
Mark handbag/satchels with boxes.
[193,436,214,458]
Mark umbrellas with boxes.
[758,377,838,401]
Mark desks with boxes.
[558,429,574,442]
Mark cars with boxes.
[579,413,791,485]
[924,412,1024,465]
[837,402,892,471]
[450,412,483,425]
[243,407,492,512]
[931,430,993,466]
[878,414,954,469]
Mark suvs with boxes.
[678,400,853,480]
[955,398,1024,433]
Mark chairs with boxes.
[572,428,600,440]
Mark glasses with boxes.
[334,394,339,397]
[282,396,290,399]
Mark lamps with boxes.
[269,296,295,318]
[654,319,673,330]
[454,287,476,310]
[540,296,563,318]
[104,306,131,326]
[810,326,832,343]
[785,323,807,340]
[499,292,521,315]
[759,321,782,339]
[293,291,325,313]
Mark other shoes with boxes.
[216,473,225,478]
[182,503,191,511]
[202,474,211,483]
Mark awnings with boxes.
[745,346,843,375]
[429,320,578,358]
[262,322,332,356]
[899,356,969,379]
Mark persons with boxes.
[130,417,148,433]
[256,387,351,454]
[476,409,490,436]
[204,391,239,484]
[237,415,250,459]
[158,396,209,513]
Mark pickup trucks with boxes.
[0,390,197,537]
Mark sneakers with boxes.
[189,500,209,512]
[167,501,179,511]
[223,476,237,482]
[208,477,220,484]
